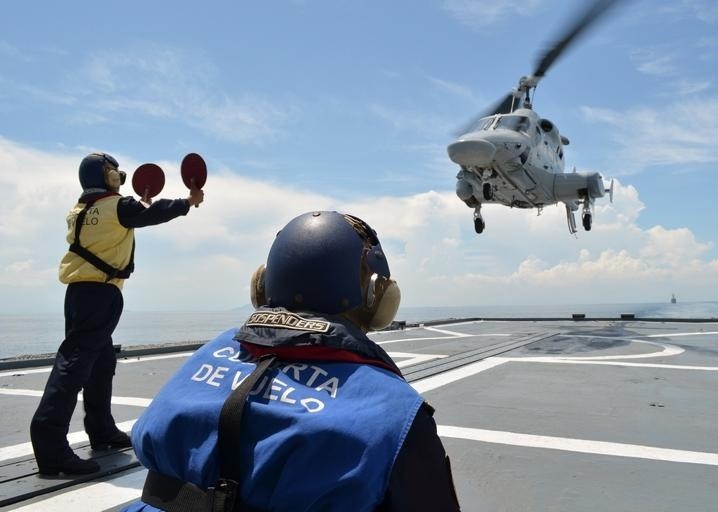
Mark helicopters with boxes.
[447,0,614,233]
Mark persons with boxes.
[30,152,204,478]
[102,210,460,512]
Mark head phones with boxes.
[249,264,402,332]
[105,167,121,188]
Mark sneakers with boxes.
[39,454,100,475]
[90,432,131,449]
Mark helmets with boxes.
[79,152,119,190]
[265,211,389,335]
